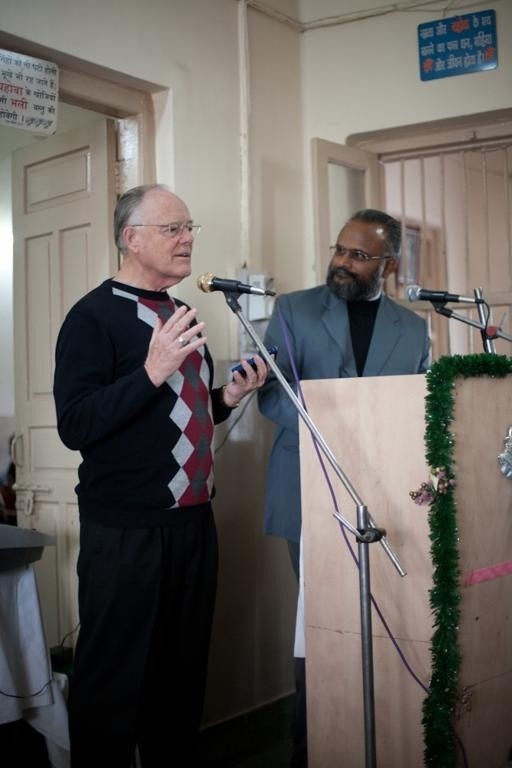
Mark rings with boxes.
[178,336,187,347]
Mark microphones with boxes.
[405,285,485,303]
[196,273,277,297]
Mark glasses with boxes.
[330,246,393,262]
[123,224,201,237]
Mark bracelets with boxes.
[218,383,241,410]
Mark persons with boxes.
[246,206,433,766]
[48,182,278,762]
